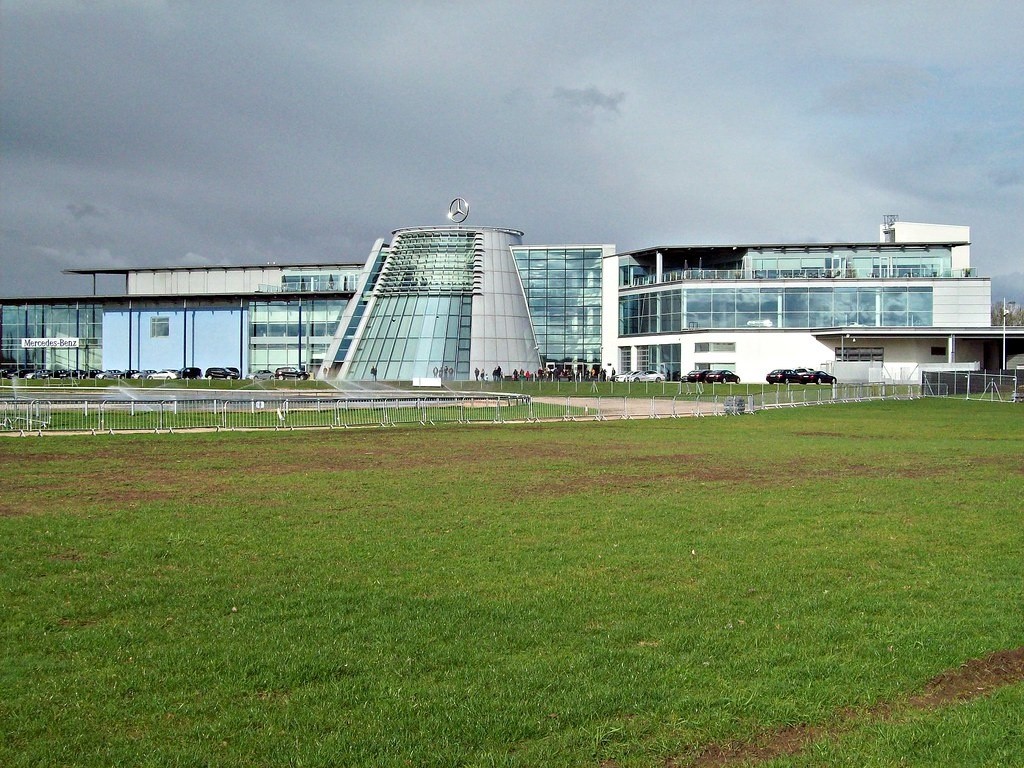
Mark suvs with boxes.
[178,367,202,380]
[205,367,240,380]
[274,367,310,380]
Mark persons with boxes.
[600,369,607,382]
[439,367,443,378]
[448,366,453,380]
[782,270,940,278]
[513,369,519,381]
[443,366,448,380]
[526,371,530,380]
[496,366,501,381]
[474,367,480,381]
[611,367,616,381]
[520,369,524,375]
[480,369,485,381]
[433,365,438,377]
[370,366,377,377]
[585,368,590,381]
[534,366,583,382]
[492,367,496,381]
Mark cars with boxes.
[624,370,665,383]
[800,371,837,385]
[0,368,104,380]
[687,370,713,383]
[1011,385,1024,402]
[113,370,140,379]
[131,370,158,379]
[147,371,181,380]
[95,370,122,380]
[245,369,274,380]
[766,369,801,385]
[705,370,740,384]
[794,368,815,376]
[614,370,642,383]
